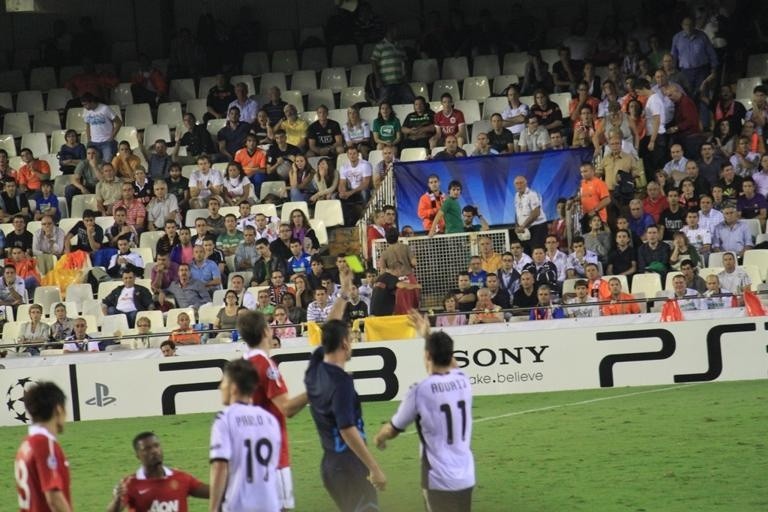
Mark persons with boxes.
[119,432,211,512]
[13,381,75,512]
[303,266,388,512]
[374,306,476,512]
[208,357,282,511]
[235,311,312,512]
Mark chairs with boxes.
[0,33,767,354]
